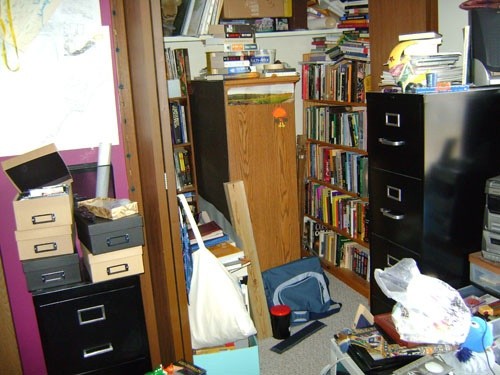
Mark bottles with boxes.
[270,304,292,339]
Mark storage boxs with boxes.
[222,0,293,19]
[3,142,146,290]
[193,333,260,375]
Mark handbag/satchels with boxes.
[260,256,342,324]
[177,194,259,352]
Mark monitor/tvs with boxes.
[468,8,500,72]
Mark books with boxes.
[301,143,369,196]
[168,101,189,145]
[305,106,368,152]
[325,0,369,29]
[205,66,299,81]
[164,47,192,97]
[378,31,470,95]
[175,194,252,357]
[299,29,371,105]
[298,217,371,283]
[171,147,193,192]
[179,0,224,37]
[296,178,371,242]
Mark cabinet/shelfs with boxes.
[163,39,199,229]
[369,88,500,317]
[32,276,152,375]
[190,72,301,273]
[299,43,372,297]
